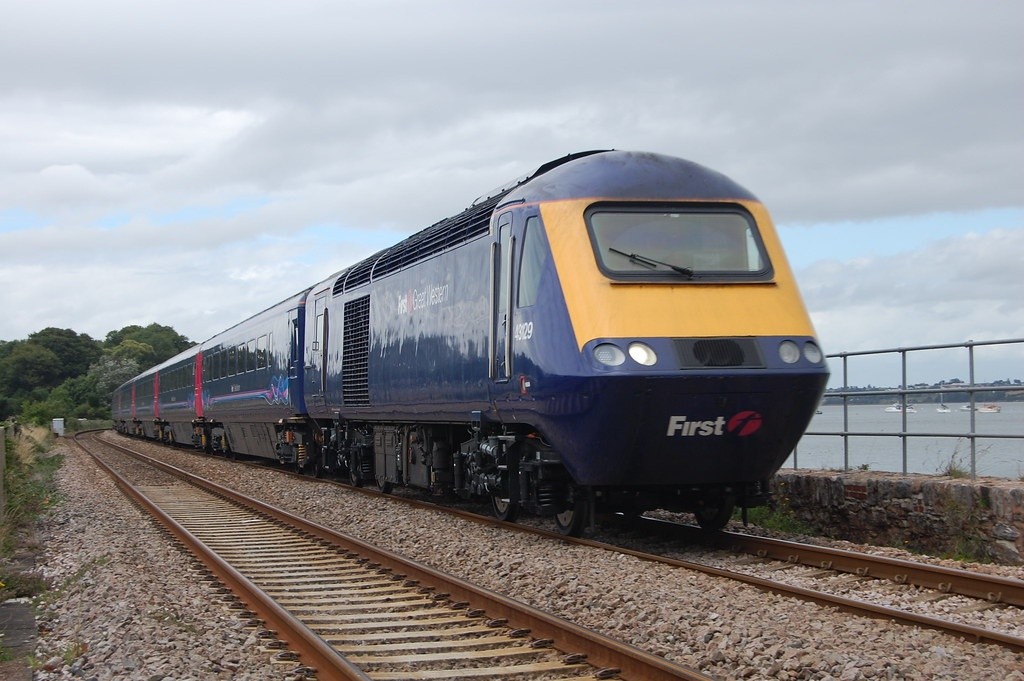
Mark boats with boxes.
[960,403,979,413]
[886,400,918,413]
[979,403,1002,412]
[937,403,950,413]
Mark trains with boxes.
[111,150,830,537]
